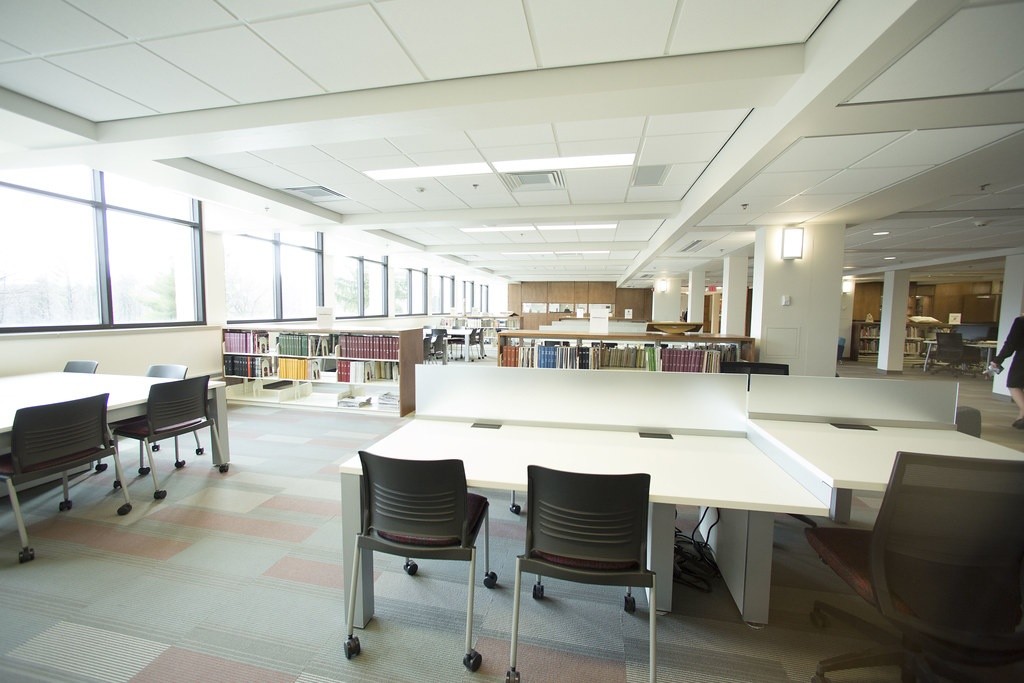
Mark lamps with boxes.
[781,227,804,260]
[660,279,667,293]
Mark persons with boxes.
[990,314,1024,430]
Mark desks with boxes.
[423,329,484,362]
[0,372,230,464]
[923,341,997,380]
[339,365,830,628]
[698,371,1024,626]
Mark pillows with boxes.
[909,316,942,323]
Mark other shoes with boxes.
[1013,417,1024,429]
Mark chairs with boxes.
[112,374,229,498]
[503,463,658,683]
[0,394,131,563]
[343,450,497,672]
[101,366,205,473]
[805,451,1024,683]
[936,333,981,377]
[64,360,108,472]
[423,328,487,364]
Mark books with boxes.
[502,342,741,373]
[337,335,399,383]
[338,392,400,408]
[439,318,517,348]
[277,334,336,381]
[859,326,924,354]
[224,333,271,377]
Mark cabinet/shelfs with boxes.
[498,330,755,374]
[508,281,652,330]
[441,316,521,344]
[221,325,423,417]
[850,321,952,360]
[703,291,723,332]
